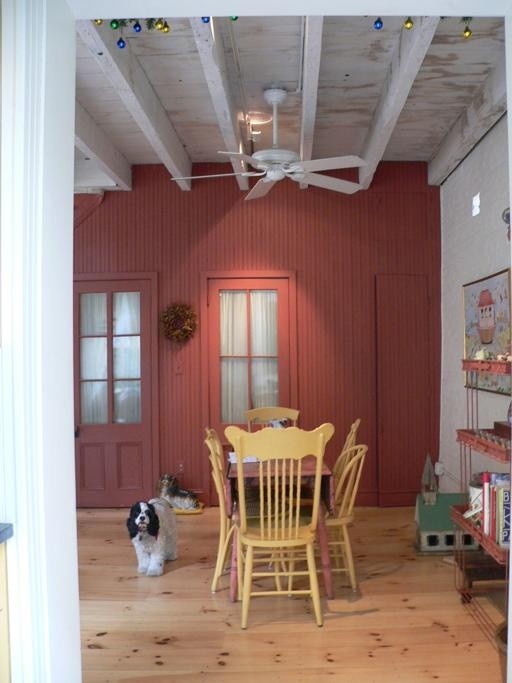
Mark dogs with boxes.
[127,498,177,576]
[160,473,199,511]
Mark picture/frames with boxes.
[461,267,512,397]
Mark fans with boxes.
[170,88,366,201]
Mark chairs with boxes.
[202,404,369,631]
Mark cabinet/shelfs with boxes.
[448,351,512,656]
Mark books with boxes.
[481,471,511,549]
